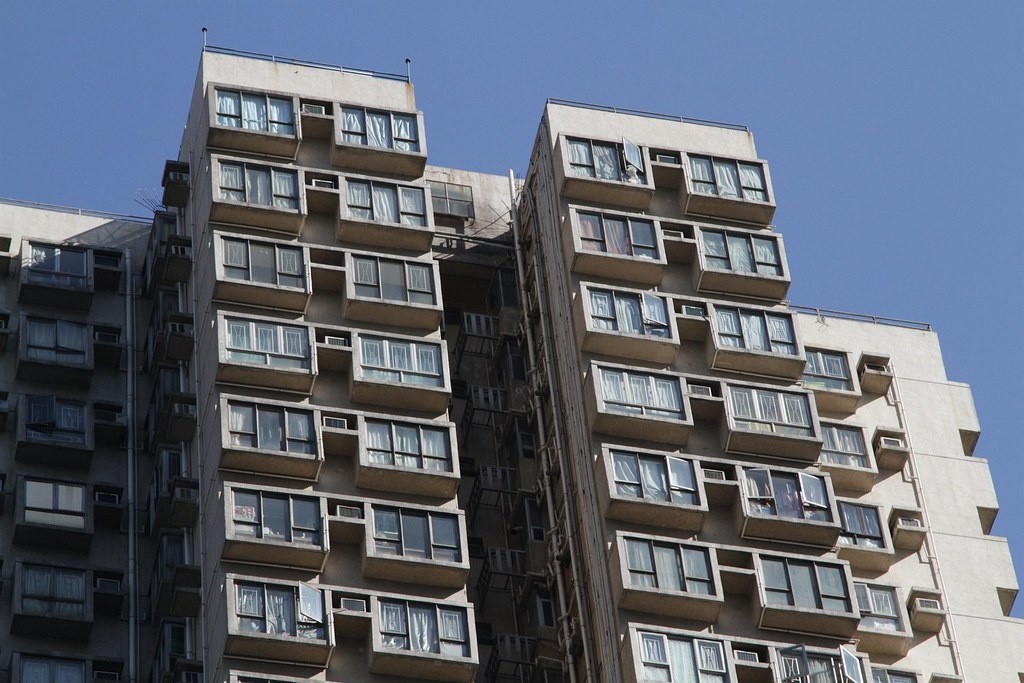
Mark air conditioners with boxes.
[337,505,362,518]
[302,103,326,115]
[166,321,203,683]
[169,245,191,255]
[312,179,334,188]
[340,597,366,612]
[689,383,713,394]
[323,415,347,429]
[168,172,190,184]
[93,671,119,681]
[95,492,119,504]
[96,332,120,345]
[660,228,685,241]
[680,305,708,316]
[96,578,120,591]
[657,154,680,164]
[735,649,760,663]
[325,336,348,346]
[862,360,945,611]
[702,467,725,481]
